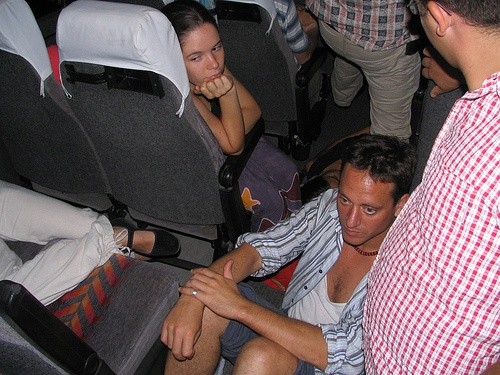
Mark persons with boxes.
[200,0,326,107]
[306,0,423,143]
[0,179,180,306]
[163,0,304,232]
[358,0,500,374]
[160,137,417,375]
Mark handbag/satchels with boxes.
[291,6,318,65]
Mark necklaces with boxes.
[354,246,378,256]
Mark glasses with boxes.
[407,0,452,16]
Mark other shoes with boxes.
[110,217,180,257]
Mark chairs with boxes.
[0,0,327,375]
